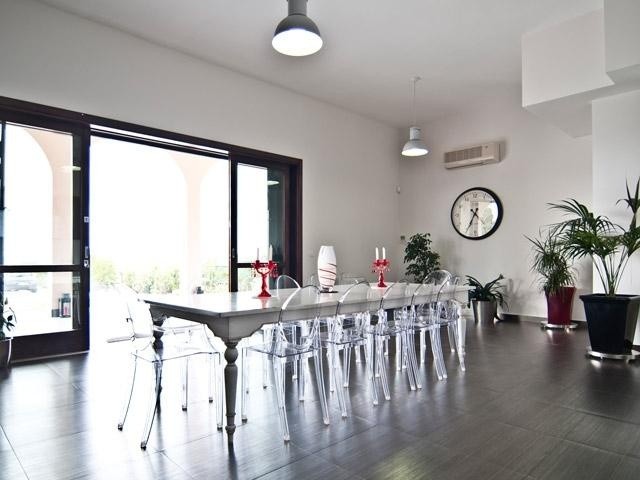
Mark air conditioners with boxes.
[442,143,500,170]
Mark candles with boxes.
[256,247,260,259]
[269,244,272,260]
[375,246,386,260]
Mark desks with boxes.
[138,280,478,450]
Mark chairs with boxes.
[386,270,451,387]
[301,282,374,418]
[396,273,459,368]
[343,278,416,403]
[243,286,330,441]
[403,276,464,379]
[116,286,225,448]
[201,275,372,402]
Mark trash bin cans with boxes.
[60,293,72,319]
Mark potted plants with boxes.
[461,272,510,328]
[522,176,640,363]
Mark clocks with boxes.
[450,187,503,240]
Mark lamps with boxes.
[269,0,324,59]
[402,75,429,157]
